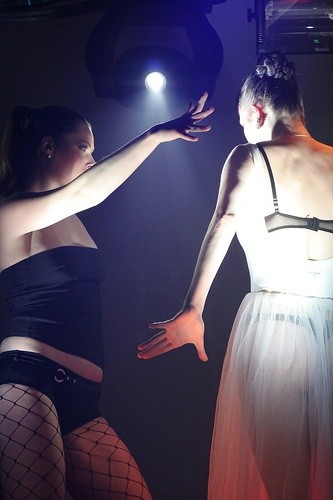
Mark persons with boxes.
[1,86,220,500]
[132,50,333,500]
